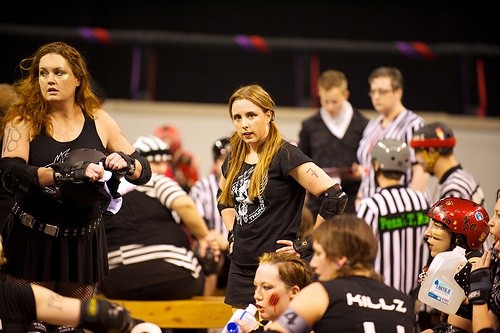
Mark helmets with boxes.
[409,121,457,148]
[133,135,172,162]
[370,139,411,174]
[427,197,490,250]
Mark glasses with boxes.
[368,86,401,97]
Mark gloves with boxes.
[75,299,133,333]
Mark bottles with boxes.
[222,303,260,333]
[418,246,470,314]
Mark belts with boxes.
[12,202,102,238]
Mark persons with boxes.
[0,42,500,333]
